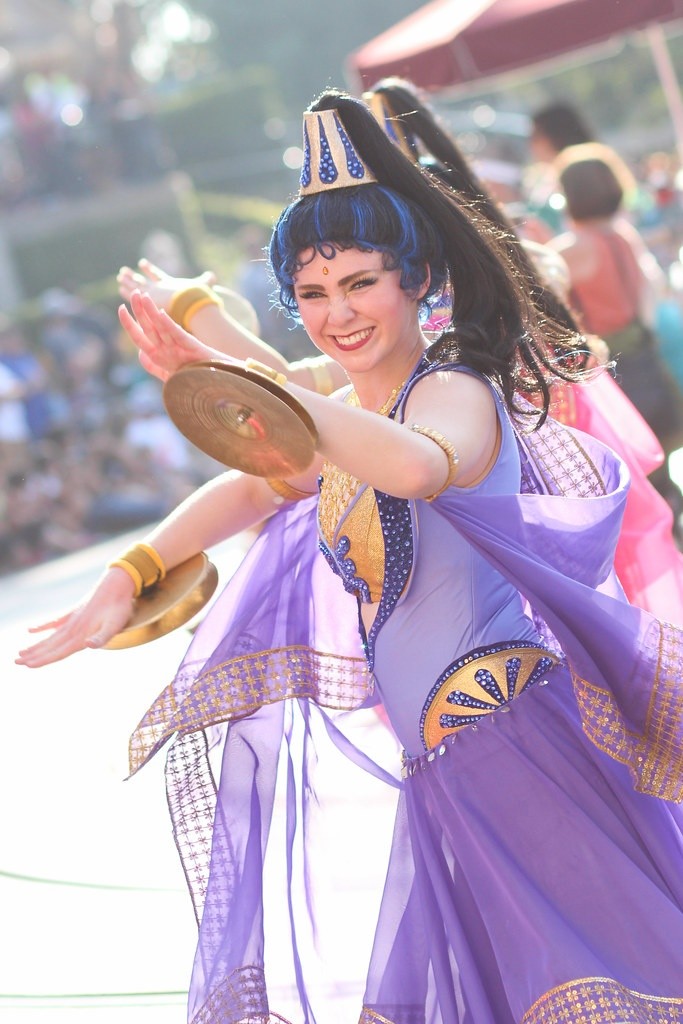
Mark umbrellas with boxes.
[342,0,683,205]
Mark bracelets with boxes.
[107,541,166,598]
[245,357,288,389]
[166,283,224,335]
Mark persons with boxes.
[13,88,683,1024]
[540,141,682,554]
[523,104,592,236]
[117,74,682,630]
[1,228,310,576]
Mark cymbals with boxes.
[89,555,219,648]
[167,362,319,475]
